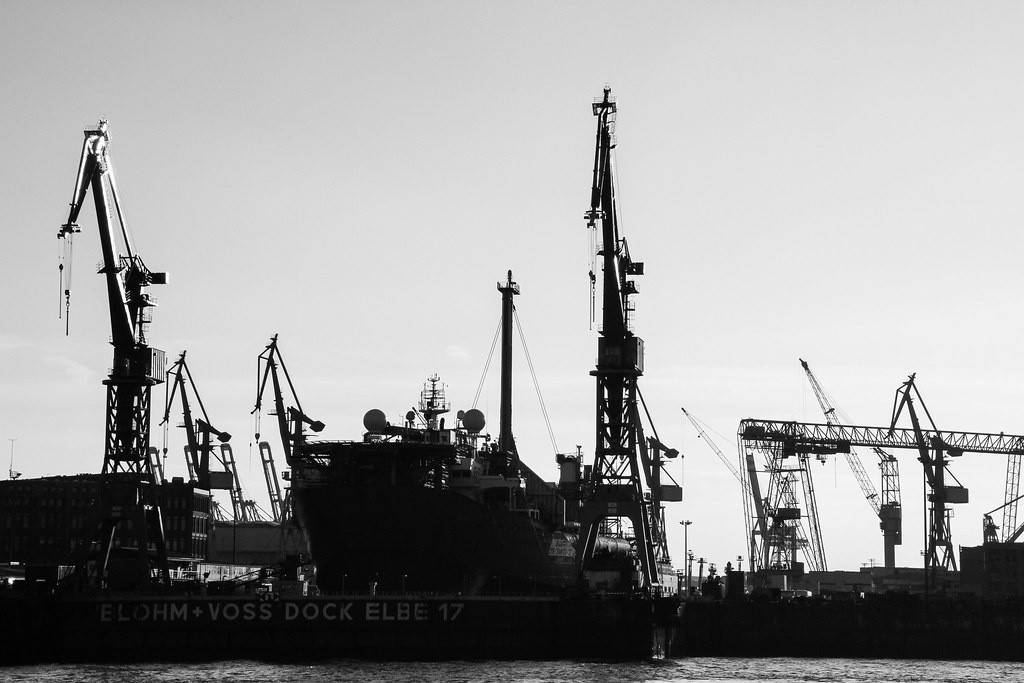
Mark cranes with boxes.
[0,72,1024,666]
